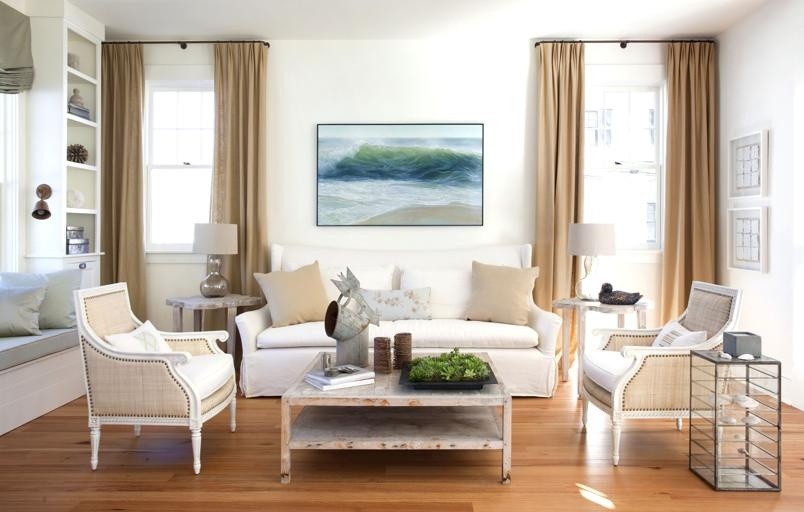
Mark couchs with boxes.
[235,242,563,399]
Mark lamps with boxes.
[31,184,51,221]
[193,223,239,297]
[568,223,617,301]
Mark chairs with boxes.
[73,282,236,475]
[580,279,743,468]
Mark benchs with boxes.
[0,265,87,438]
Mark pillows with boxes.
[0,273,47,337]
[39,266,84,329]
[103,319,173,355]
[651,319,707,348]
[462,260,540,325]
[252,260,332,327]
[356,287,433,320]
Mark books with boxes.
[302,363,377,391]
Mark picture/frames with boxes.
[729,130,769,197]
[727,207,767,274]
[316,123,484,227]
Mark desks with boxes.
[551,296,647,400]
[164,293,263,364]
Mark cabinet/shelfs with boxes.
[689,350,781,493]
[23,0,104,287]
